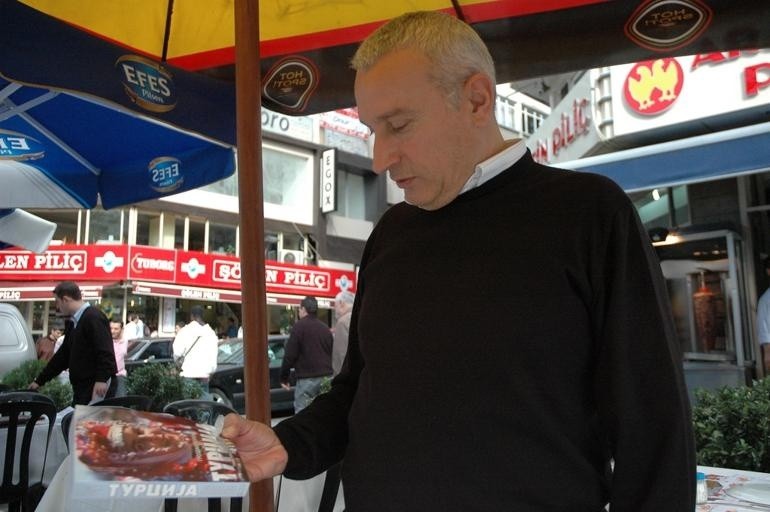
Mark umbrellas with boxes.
[0,0,770,512]
[0,209,57,254]
[0,78,237,212]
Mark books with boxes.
[68,404,250,499]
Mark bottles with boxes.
[696,472,708,504]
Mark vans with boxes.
[0,303,38,385]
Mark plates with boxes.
[723,482,770,505]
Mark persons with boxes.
[78,423,208,478]
[279,297,334,415]
[756,255,770,377]
[175,322,185,334]
[226,317,237,339]
[27,282,118,409]
[331,290,355,378]
[35,311,144,376]
[221,11,696,512]
[172,306,218,423]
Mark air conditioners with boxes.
[281,249,304,266]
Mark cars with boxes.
[124,335,296,414]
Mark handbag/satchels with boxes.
[176,356,185,370]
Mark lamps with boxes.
[649,227,669,243]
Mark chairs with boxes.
[0,390,242,512]
